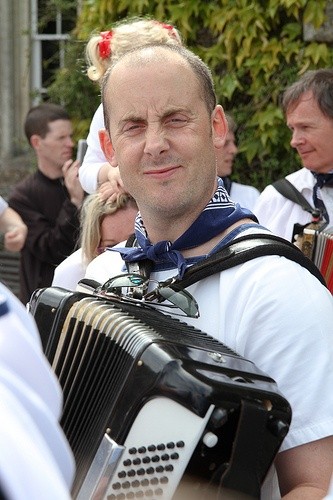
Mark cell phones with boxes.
[76,139,88,165]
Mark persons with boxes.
[77,43,333,500]
[0,17,261,500]
[252,68,333,245]
